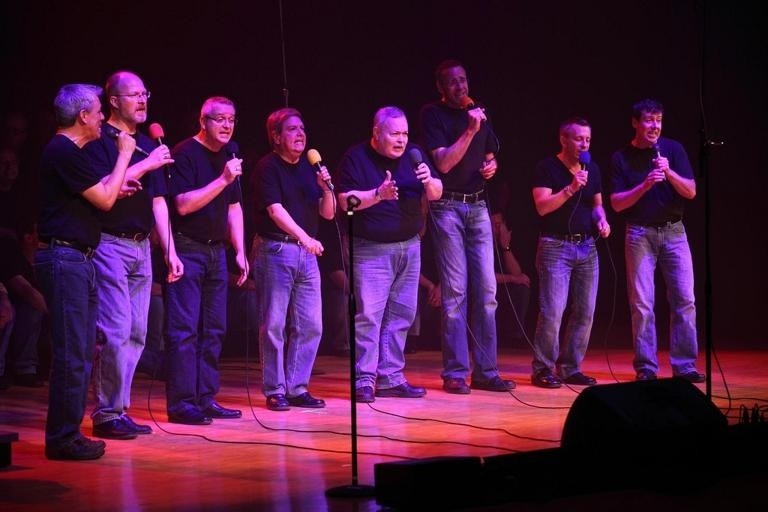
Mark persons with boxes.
[0,100,52,194]
[228,246,259,362]
[0,283,15,390]
[334,106,442,403]
[605,99,709,382]
[168,96,250,426]
[33,85,144,460]
[316,218,354,359]
[531,117,610,388]
[404,241,444,353]
[137,232,170,372]
[0,217,50,386]
[491,206,530,350]
[254,106,338,412]
[412,59,515,394]
[86,70,183,440]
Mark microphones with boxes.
[579,152,591,190]
[150,123,172,180]
[225,139,240,184]
[459,95,488,127]
[408,148,424,185]
[652,143,666,181]
[307,149,334,190]
[106,127,150,157]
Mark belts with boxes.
[56,240,95,258]
[202,238,217,246]
[564,232,590,242]
[442,190,484,203]
[641,216,682,227]
[261,234,302,246]
[106,230,149,242]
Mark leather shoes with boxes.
[92,420,136,440]
[167,409,213,425]
[531,372,560,388]
[355,386,375,403]
[471,376,515,390]
[678,370,705,383]
[46,442,105,462]
[13,374,43,387]
[121,414,152,434]
[205,403,241,419]
[442,377,471,394]
[561,372,596,385]
[634,370,656,381]
[288,391,324,407]
[75,433,106,448]
[266,393,291,411]
[374,381,426,398]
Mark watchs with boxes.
[502,245,511,251]
[376,188,383,202]
[564,186,574,197]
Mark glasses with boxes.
[205,116,236,125]
[114,90,151,100]
